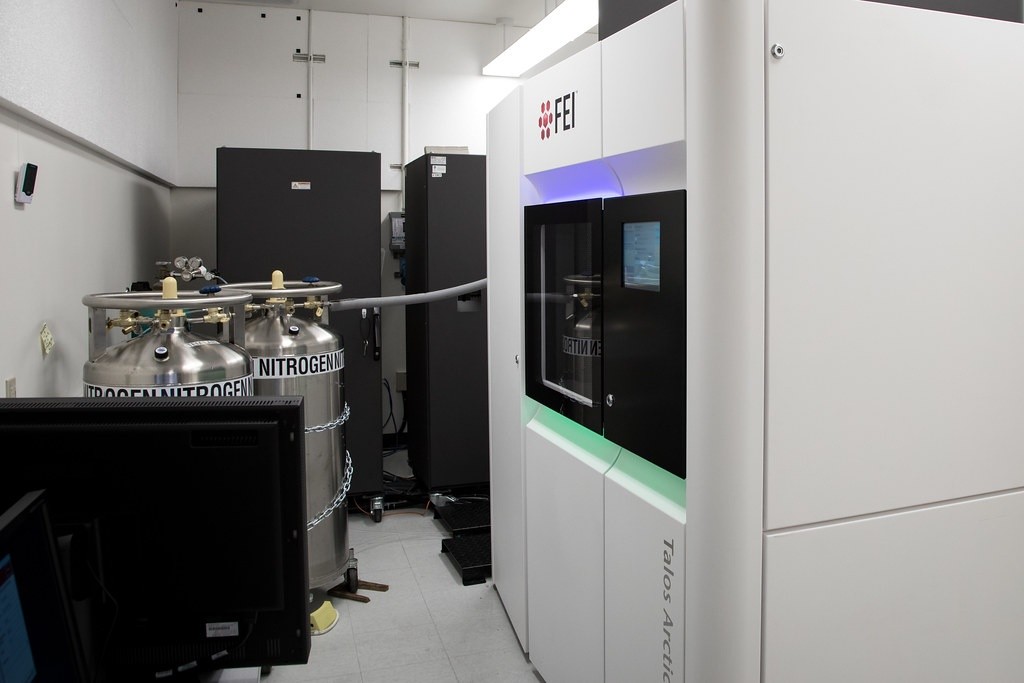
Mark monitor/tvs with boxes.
[0,397,312,683]
[0,489,91,683]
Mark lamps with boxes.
[481,0,599,78]
